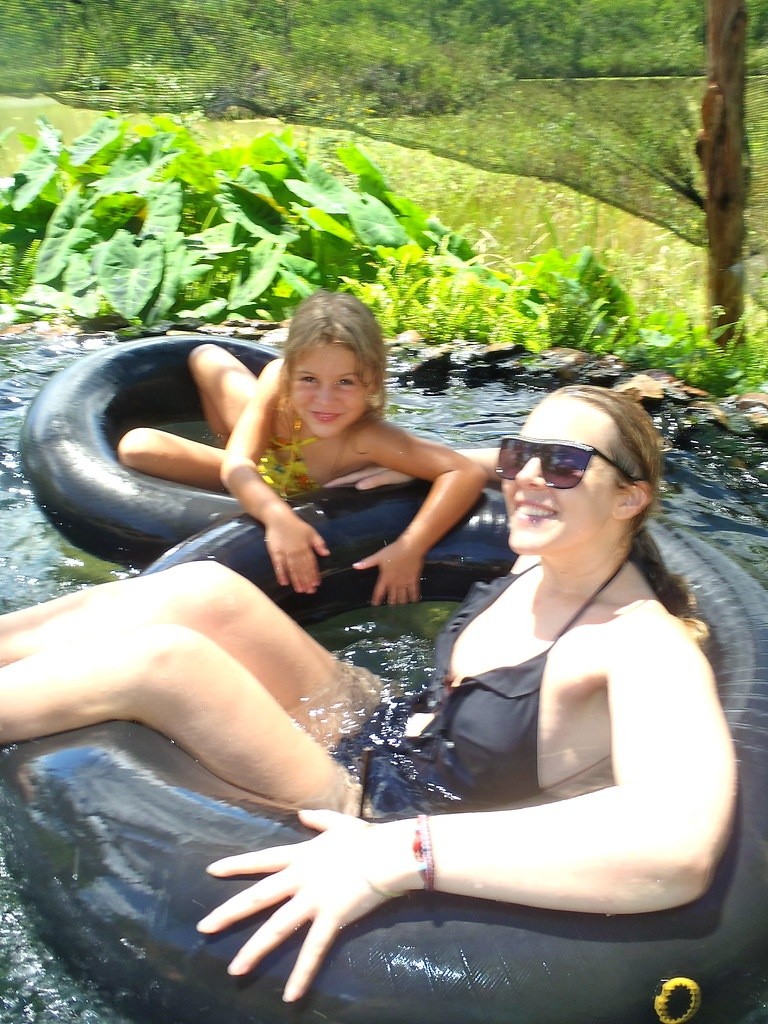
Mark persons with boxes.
[119,293,487,604]
[1,385,738,1001]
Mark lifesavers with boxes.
[20,332,288,569]
[0,468,768,1024]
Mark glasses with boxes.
[495,433,635,491]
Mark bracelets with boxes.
[413,812,434,891]
